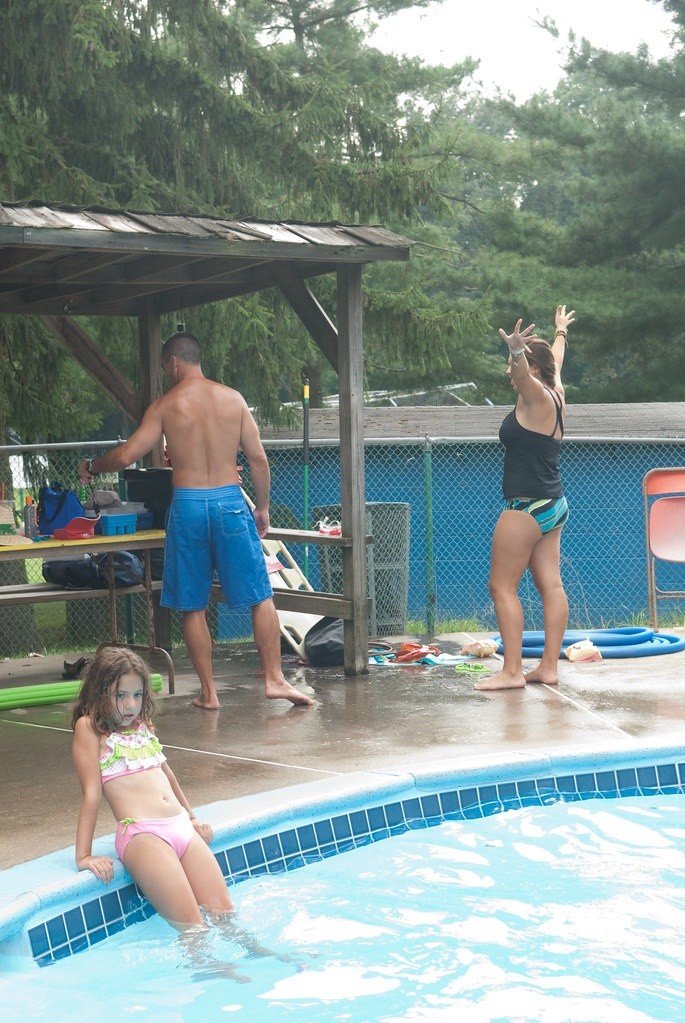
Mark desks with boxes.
[0,529,175,696]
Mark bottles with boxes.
[25,496,37,539]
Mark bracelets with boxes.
[508,345,525,367]
[555,330,567,338]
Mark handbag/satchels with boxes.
[305,617,393,667]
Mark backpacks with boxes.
[41,549,143,587]
[36,480,85,535]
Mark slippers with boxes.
[455,662,490,671]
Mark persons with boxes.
[72,648,235,923]
[80,333,315,709]
[474,304,576,690]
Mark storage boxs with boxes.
[85,513,138,536]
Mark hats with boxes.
[0,504,34,545]
[53,515,102,538]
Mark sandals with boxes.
[63,658,94,678]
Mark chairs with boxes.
[641,466,685,633]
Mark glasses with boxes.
[319,527,342,537]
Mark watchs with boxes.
[86,459,99,476]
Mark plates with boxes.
[0,535,34,545]
